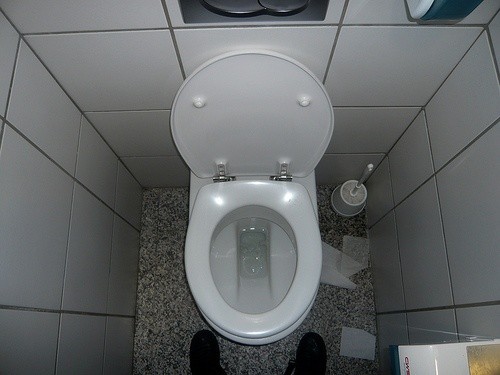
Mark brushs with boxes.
[342,164,373,204]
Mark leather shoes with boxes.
[295,331,327,375]
[190,329,227,374]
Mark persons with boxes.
[190,329,327,375]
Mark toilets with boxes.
[170,48,335,345]
